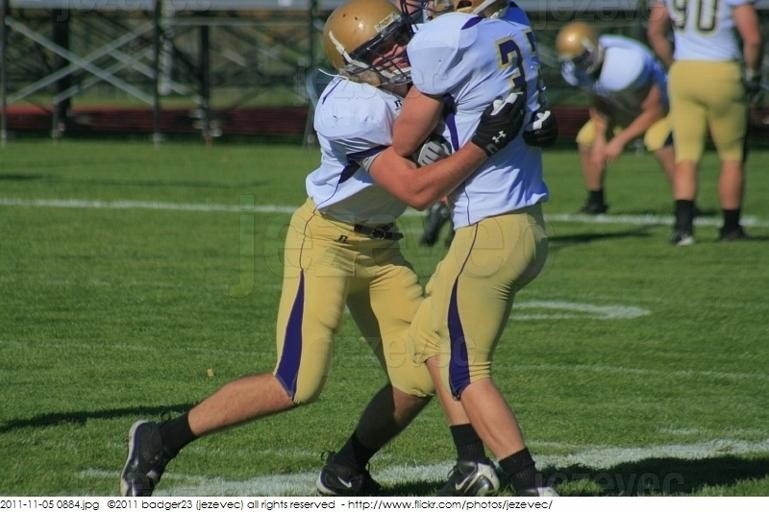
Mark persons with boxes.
[647,0,763,245]
[394,0,549,496]
[556,20,700,217]
[394,0,454,248]
[120,0,557,496]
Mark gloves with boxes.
[740,74,762,102]
[471,87,526,158]
[412,136,445,168]
[522,106,560,147]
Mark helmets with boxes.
[323,0,417,88]
[554,22,601,86]
[433,0,509,18]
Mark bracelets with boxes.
[745,69,762,82]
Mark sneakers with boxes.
[435,457,500,496]
[315,450,389,496]
[118,417,167,497]
[570,202,609,218]
[719,224,754,242]
[421,200,450,248]
[665,229,695,246]
[517,485,559,497]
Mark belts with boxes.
[353,223,403,242]
